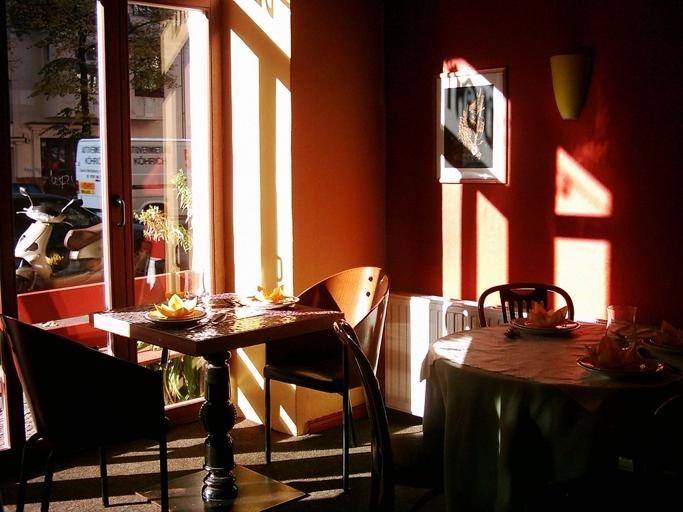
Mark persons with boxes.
[137,144,165,276]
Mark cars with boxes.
[14,192,148,274]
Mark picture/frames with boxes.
[432,67,511,186]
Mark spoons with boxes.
[186,314,227,331]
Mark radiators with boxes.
[380,290,529,419]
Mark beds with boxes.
[0,311,176,512]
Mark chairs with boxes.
[332,315,425,511]
[476,282,574,327]
[263,262,388,491]
[636,392,680,512]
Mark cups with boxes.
[604,305,637,351]
[183,271,205,299]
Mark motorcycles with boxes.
[14,185,153,294]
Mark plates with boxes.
[577,336,683,375]
[243,292,301,309]
[507,318,583,335]
[145,308,207,322]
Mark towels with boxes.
[154,292,199,318]
[257,283,284,303]
[582,336,640,368]
[521,298,568,327]
[650,322,682,348]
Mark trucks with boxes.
[74,137,190,227]
